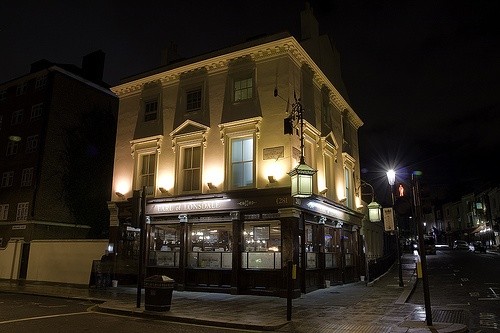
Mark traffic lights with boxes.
[397,183,410,202]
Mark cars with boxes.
[452,240,469,250]
[412,240,417,249]
[474,241,487,253]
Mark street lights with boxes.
[384,166,405,287]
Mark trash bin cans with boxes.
[144,275,176,311]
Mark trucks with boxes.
[417,235,437,255]
